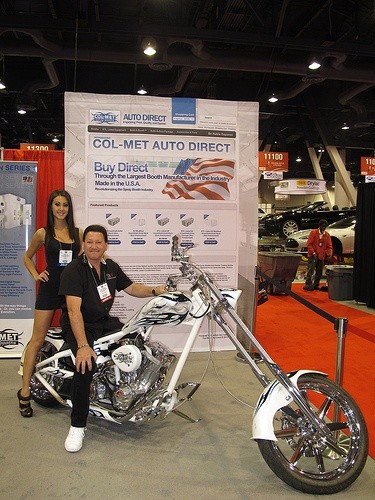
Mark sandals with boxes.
[17,388,34,418]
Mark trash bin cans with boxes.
[258,251,302,294]
[325,264,354,300]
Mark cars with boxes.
[257,208,267,219]
[285,216,355,256]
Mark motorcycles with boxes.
[18,235,369,494]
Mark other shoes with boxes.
[314,287,318,290]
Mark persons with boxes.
[302,219,333,291]
[17,190,107,417]
[59,224,173,453]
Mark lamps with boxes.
[268,90,278,103]
[51,134,59,143]
[308,56,321,70]
[137,84,147,94]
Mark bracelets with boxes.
[152,287,160,296]
[77,344,89,350]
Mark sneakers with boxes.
[64,425,87,453]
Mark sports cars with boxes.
[259,201,356,239]
